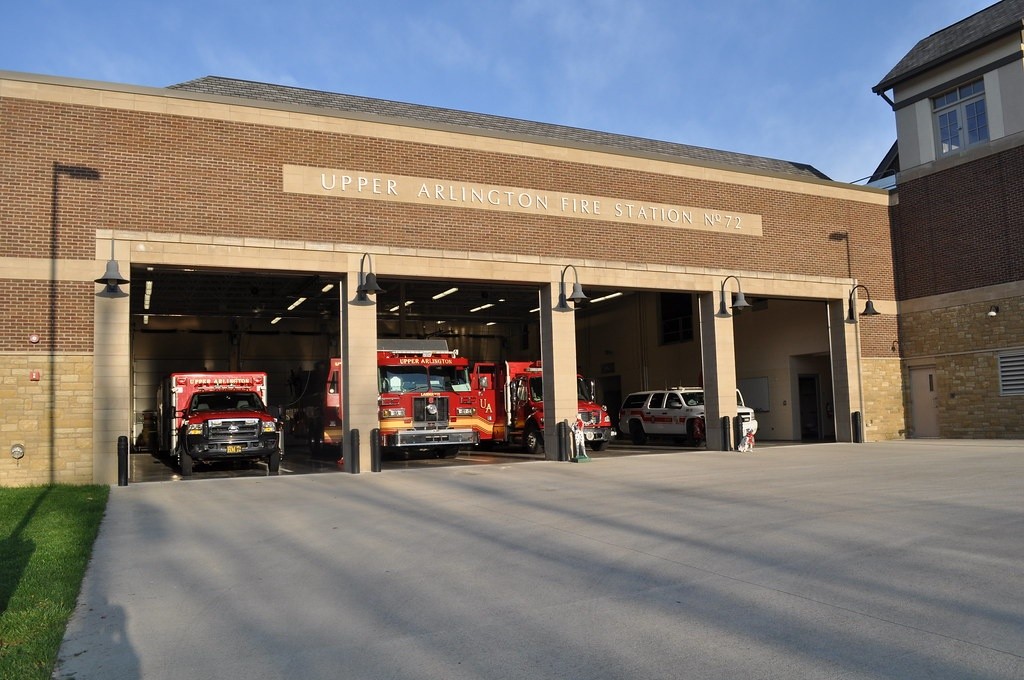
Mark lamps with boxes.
[849,285,881,320]
[94,239,131,292]
[356,252,386,301]
[720,276,752,314]
[559,265,587,308]
[988,306,999,317]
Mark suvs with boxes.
[617,385,759,448]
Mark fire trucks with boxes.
[471,359,613,455]
[307,336,481,461]
[155,372,287,478]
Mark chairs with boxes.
[198,404,209,409]
[237,400,249,408]
